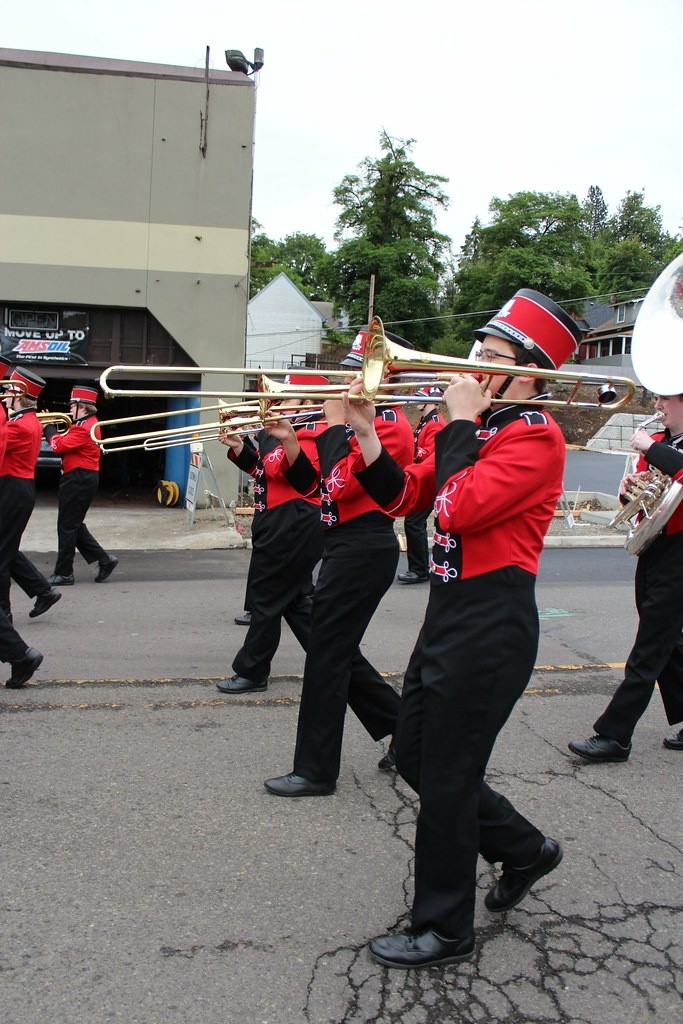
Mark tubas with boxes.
[7,407,76,443]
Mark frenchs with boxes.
[606,250,683,559]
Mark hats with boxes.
[414,385,444,406]
[284,366,330,423]
[68,385,98,419]
[473,288,584,406]
[340,325,414,382]
[1,366,46,406]
[0,355,12,380]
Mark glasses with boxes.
[475,349,518,363]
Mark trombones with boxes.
[99,315,639,413]
[88,397,330,456]
[0,380,29,398]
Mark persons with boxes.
[38,385,119,586]
[568,393,683,762]
[215,287,583,967]
[0,355,65,687]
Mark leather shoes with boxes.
[568,735,632,762]
[28,586,61,618]
[264,771,336,797]
[94,555,118,583]
[484,835,563,911]
[233,612,252,625]
[663,729,683,750]
[5,647,43,687]
[215,674,267,692]
[367,927,474,972]
[398,569,429,582]
[378,732,404,769]
[46,573,74,585]
